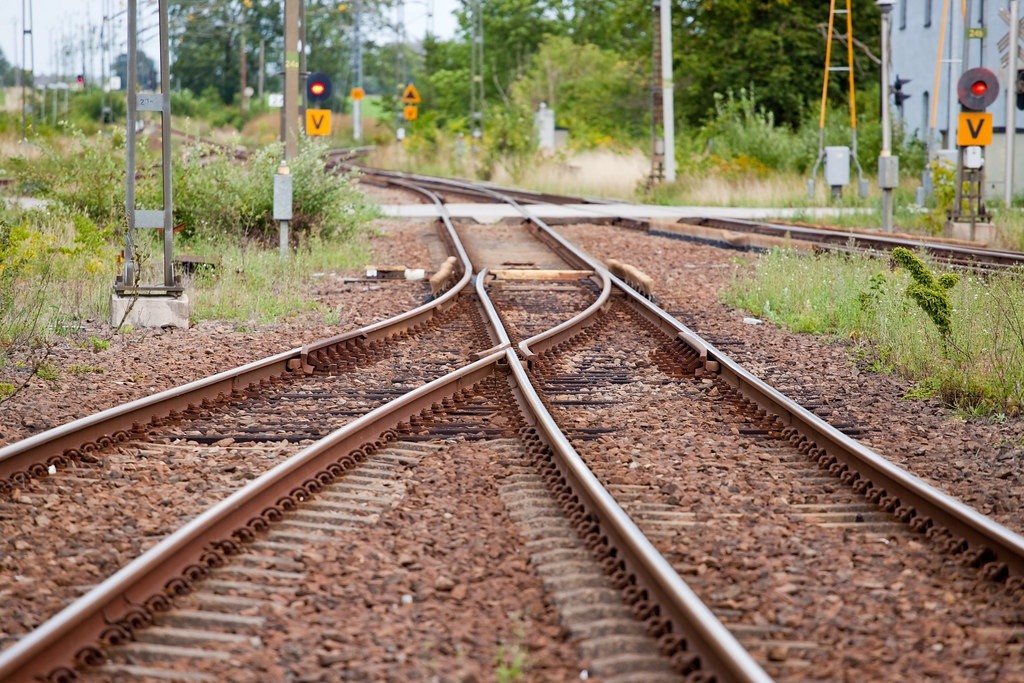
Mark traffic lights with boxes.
[959,68,999,110]
[304,72,332,102]
